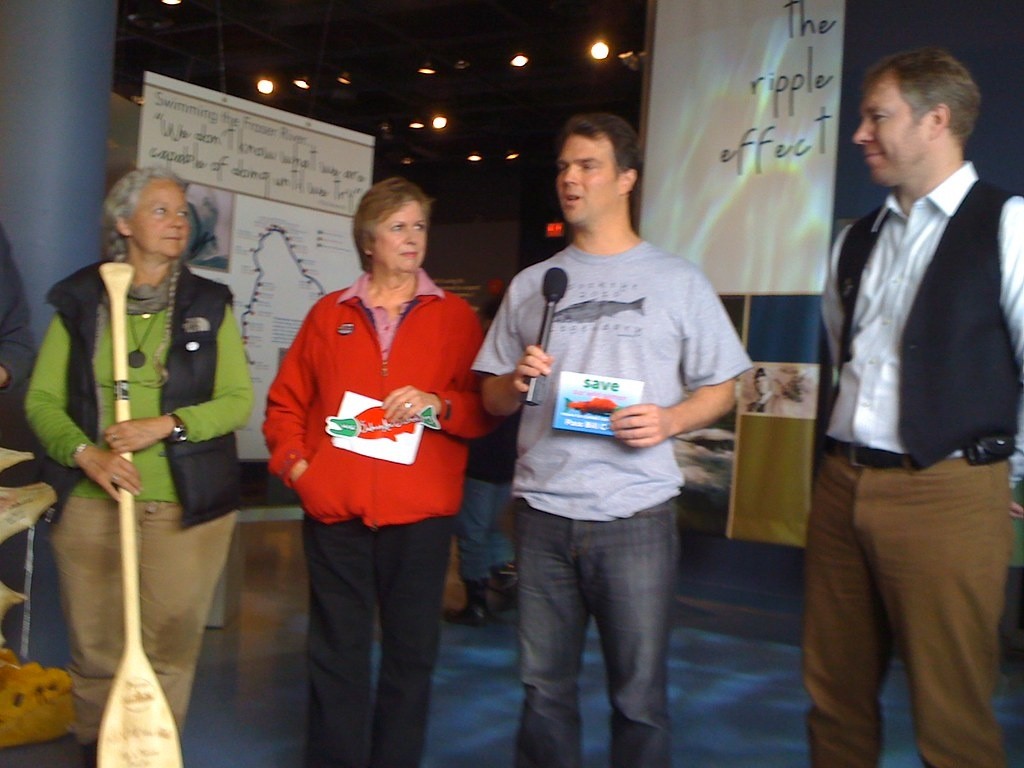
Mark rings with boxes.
[112,432,117,441]
[111,473,120,483]
[404,402,413,409]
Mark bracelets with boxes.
[71,444,88,458]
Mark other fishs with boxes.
[564,397,623,415]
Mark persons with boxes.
[473,112,753,768]
[23,170,253,767]
[0,223,37,391]
[803,49,1024,768]
[263,179,498,768]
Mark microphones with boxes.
[523,267,568,406]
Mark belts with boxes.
[821,434,966,470]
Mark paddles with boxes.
[94,261,186,767]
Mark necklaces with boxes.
[130,314,157,368]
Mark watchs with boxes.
[164,414,188,442]
